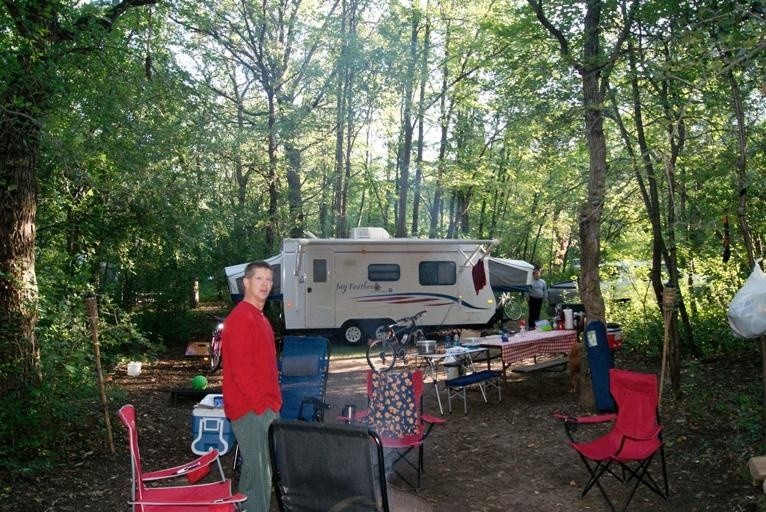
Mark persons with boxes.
[525,267,548,331]
[221,259,283,512]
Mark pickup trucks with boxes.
[547,259,717,319]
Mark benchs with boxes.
[512,357,568,393]
[444,370,503,415]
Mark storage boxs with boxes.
[191,393,239,454]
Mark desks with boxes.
[464,327,578,402]
[411,345,489,413]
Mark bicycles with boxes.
[499,296,522,321]
[365,310,429,373]
[204,311,226,374]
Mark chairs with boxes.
[119,402,248,512]
[556,304,588,334]
[551,369,670,511]
[266,418,391,512]
[277,336,332,422]
[337,368,450,491]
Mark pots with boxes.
[417,339,438,354]
[606,323,623,350]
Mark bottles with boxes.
[519,320,527,334]
[553,307,586,331]
[501,325,508,341]
[443,333,460,349]
[346,404,357,424]
